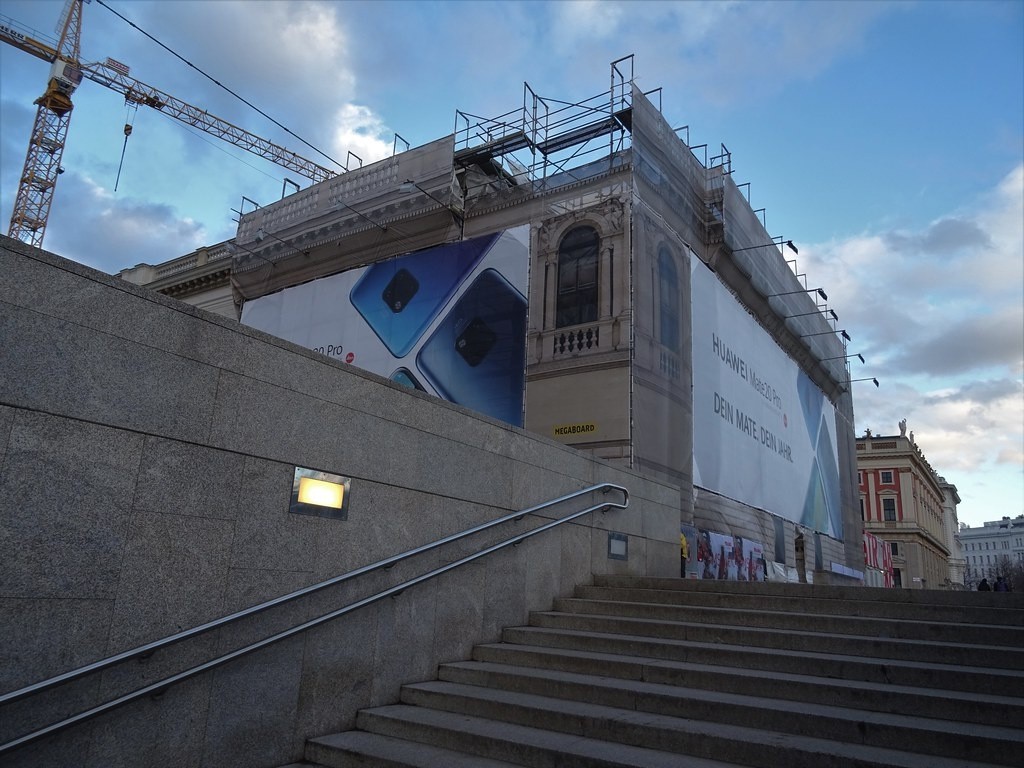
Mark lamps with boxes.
[800,330,850,341]
[768,288,827,301]
[225,241,276,267]
[731,241,798,255]
[819,353,864,364]
[838,378,878,388]
[399,179,463,228]
[785,310,838,322]
[255,228,308,257]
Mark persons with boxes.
[994,577,1009,592]
[681,532,687,578]
[977,579,990,591]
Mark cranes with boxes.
[0,0,343,254]
